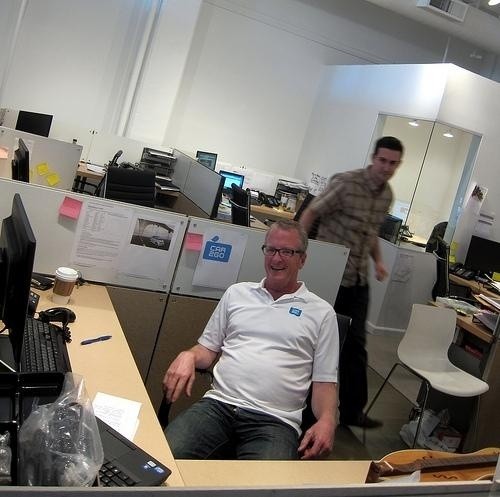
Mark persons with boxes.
[300,136,403,429]
[163,217,339,460]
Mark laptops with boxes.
[88,414,173,487]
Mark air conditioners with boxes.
[416,0,470,23]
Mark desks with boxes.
[402,234,428,247]
[0,275,185,489]
[175,457,382,491]
[219,202,295,221]
[72,161,180,198]
[409,270,500,452]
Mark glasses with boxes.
[262,245,304,257]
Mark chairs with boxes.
[229,183,251,227]
[73,150,156,208]
[433,236,475,305]
[362,303,488,450]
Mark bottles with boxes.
[286,194,297,213]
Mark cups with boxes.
[53,267,79,305]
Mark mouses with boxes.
[39,307,76,323]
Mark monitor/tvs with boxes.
[15,110,54,138]
[0,193,36,368]
[11,138,29,183]
[463,235,500,280]
[196,150,217,171]
[219,170,245,197]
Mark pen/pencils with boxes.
[80,335,112,345]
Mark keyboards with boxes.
[21,313,72,375]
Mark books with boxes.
[147,152,180,191]
[473,279,500,335]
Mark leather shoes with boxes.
[339,411,382,429]
